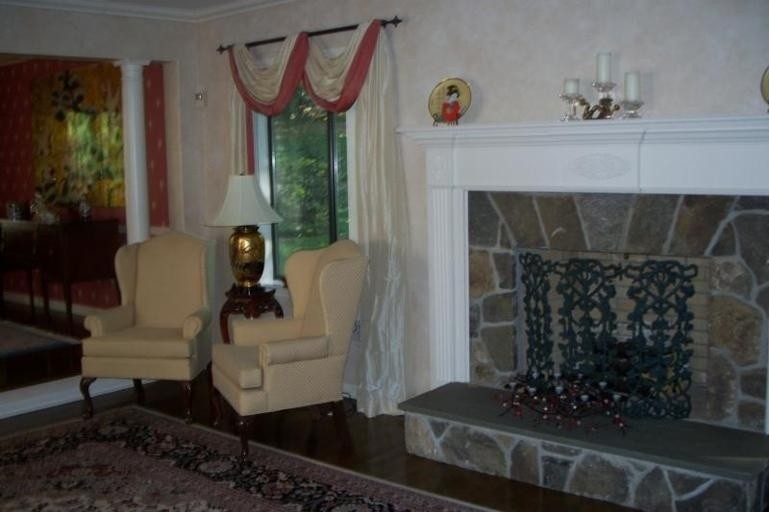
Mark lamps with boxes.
[205,173,284,292]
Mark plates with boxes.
[760,66,769,107]
[427,77,471,122]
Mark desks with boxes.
[0,219,41,304]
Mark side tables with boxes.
[220,288,284,344]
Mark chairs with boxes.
[78,232,218,425]
[211,240,359,463]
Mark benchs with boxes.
[26,220,121,322]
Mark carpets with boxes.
[1,405,500,511]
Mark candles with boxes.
[565,79,578,92]
[624,73,642,102]
[596,53,616,83]
